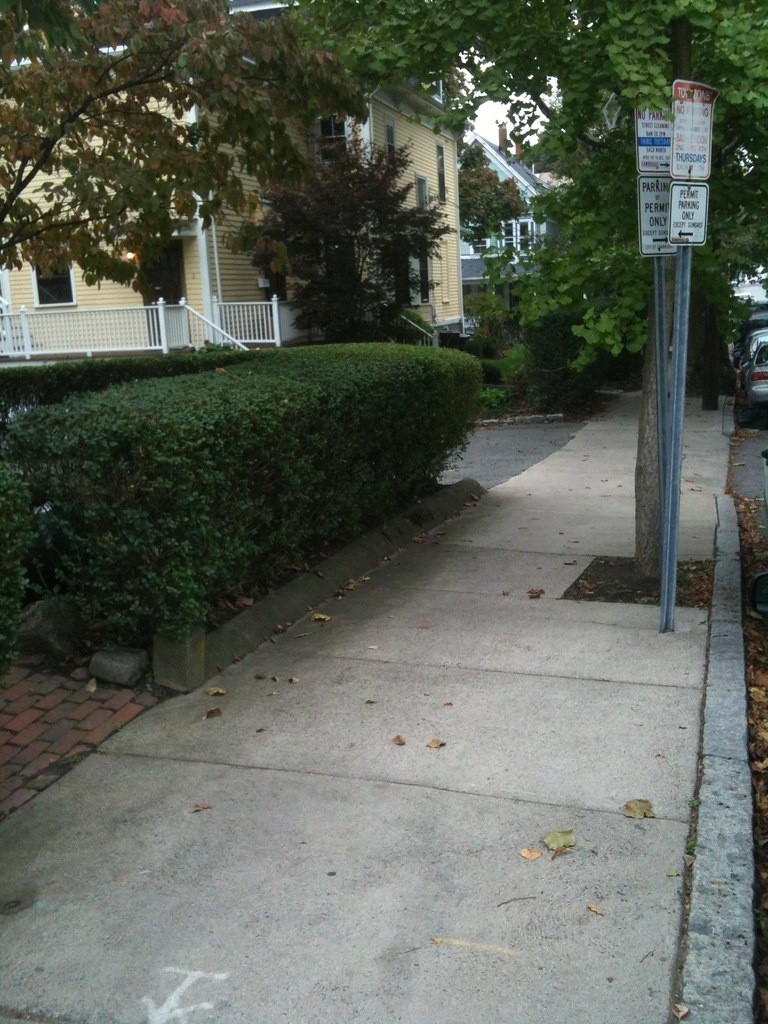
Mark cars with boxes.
[731,304,768,414]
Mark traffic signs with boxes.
[637,174,678,258]
[667,181,709,246]
